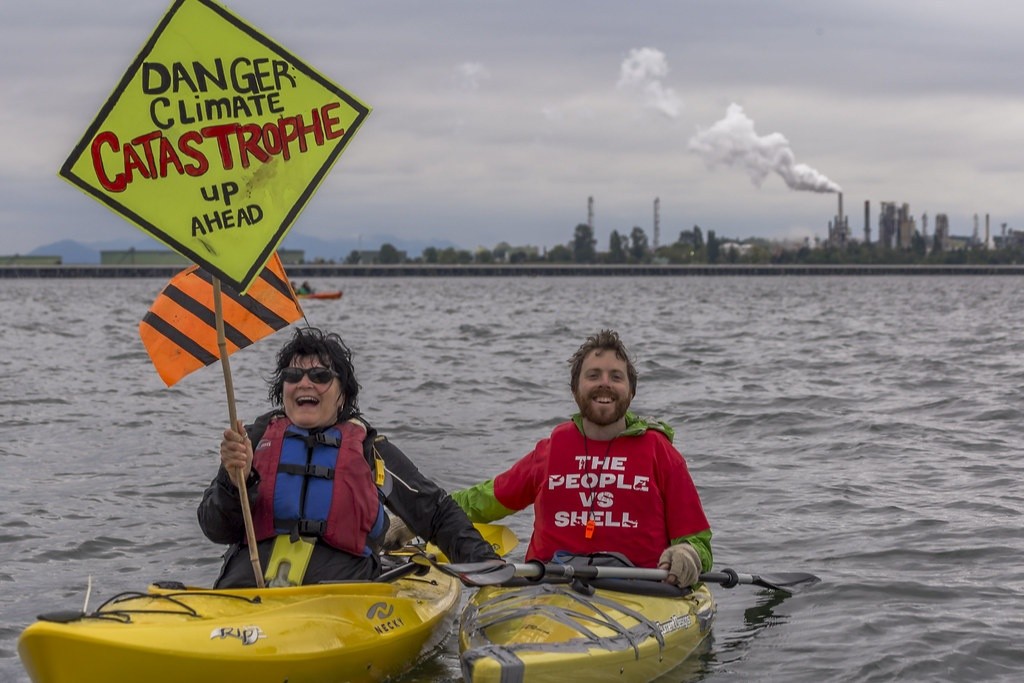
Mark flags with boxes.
[138,248,302,390]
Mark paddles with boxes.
[434,555,822,597]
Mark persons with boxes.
[382,329,713,589]
[191,323,508,588]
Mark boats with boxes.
[454,567,715,682]
[17,546,460,683]
[296,290,343,299]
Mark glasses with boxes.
[280,367,339,384]
